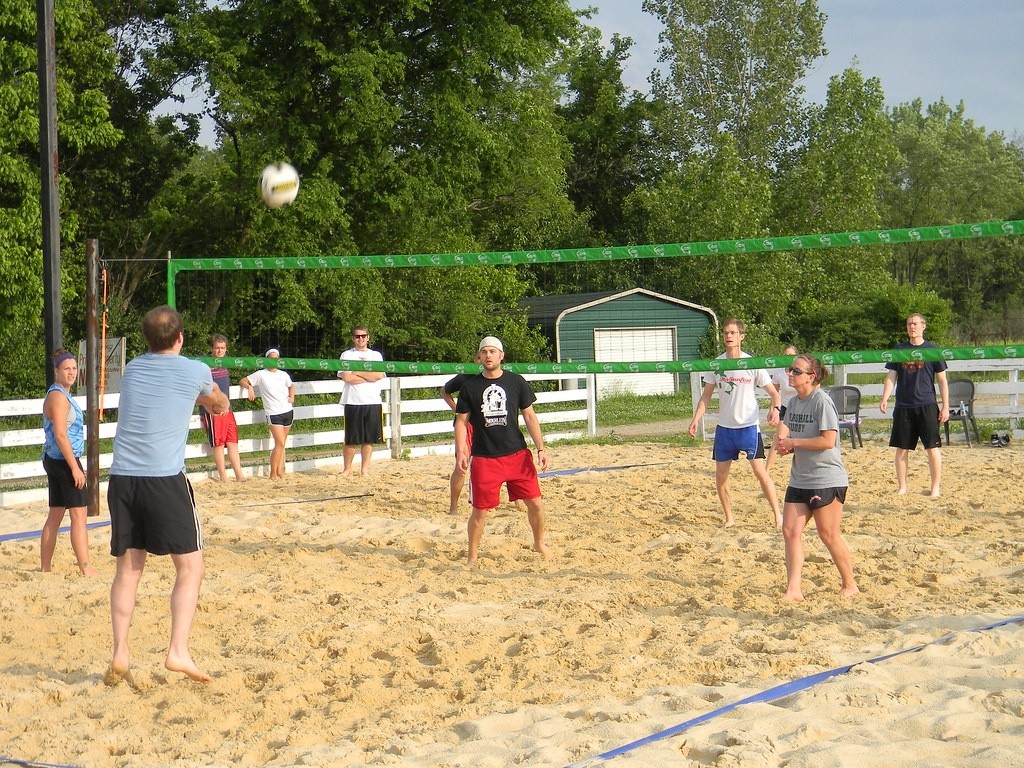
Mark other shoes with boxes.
[1001,433,1011,445]
[990,434,999,443]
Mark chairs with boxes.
[827,386,864,449]
[944,379,981,449]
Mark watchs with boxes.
[774,405,782,411]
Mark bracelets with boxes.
[538,450,542,452]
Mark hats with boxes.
[479,336,503,353]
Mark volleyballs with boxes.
[256,160,302,210]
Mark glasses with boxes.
[353,334,367,338]
[789,366,815,377]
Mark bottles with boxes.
[960,400,965,417]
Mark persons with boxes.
[441,373,524,514]
[196,334,247,483]
[880,313,949,496]
[775,354,859,600]
[41,348,97,575]
[689,318,784,530]
[765,345,799,472]
[455,336,548,564]
[107,304,230,682]
[337,326,387,477]
[238,347,295,479]
